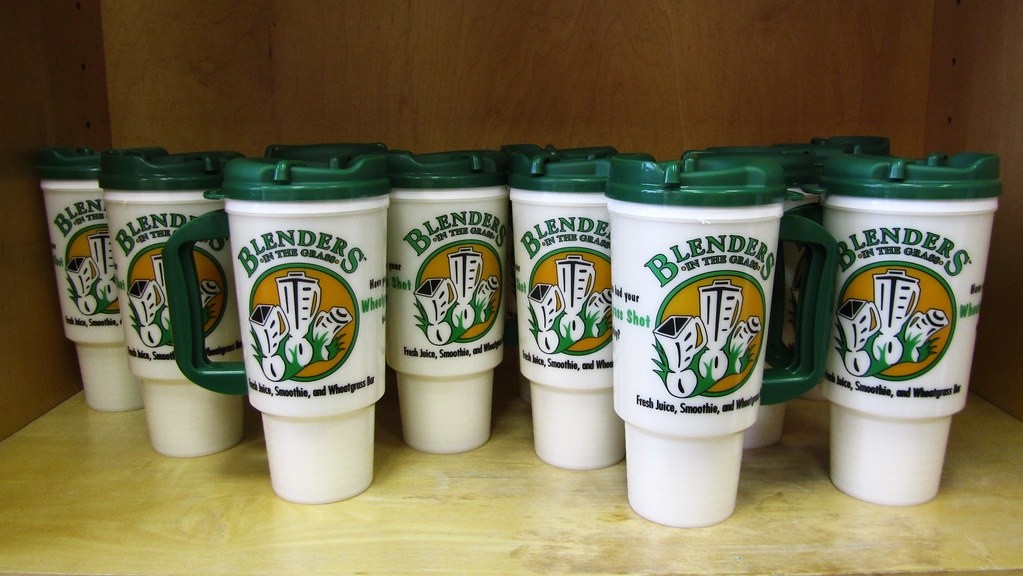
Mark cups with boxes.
[506,142,625,473]
[809,147,1004,508]
[681,143,825,456]
[38,145,143,416]
[603,146,845,532]
[161,142,397,506]
[95,144,251,455]
[381,147,510,458]
